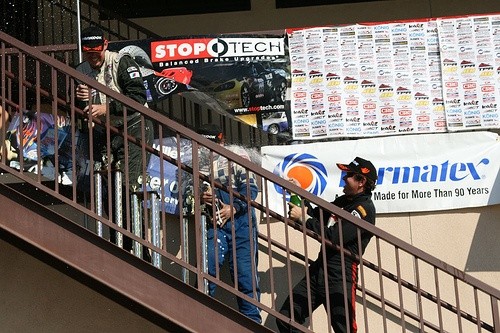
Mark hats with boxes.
[336,156,376,181]
[81,27,106,51]
[197,124,223,143]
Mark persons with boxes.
[275,156,378,333]
[0,105,76,200]
[68,26,152,265]
[181,125,263,326]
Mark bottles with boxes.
[289,178,302,211]
[202,186,224,227]
[76,83,90,134]
